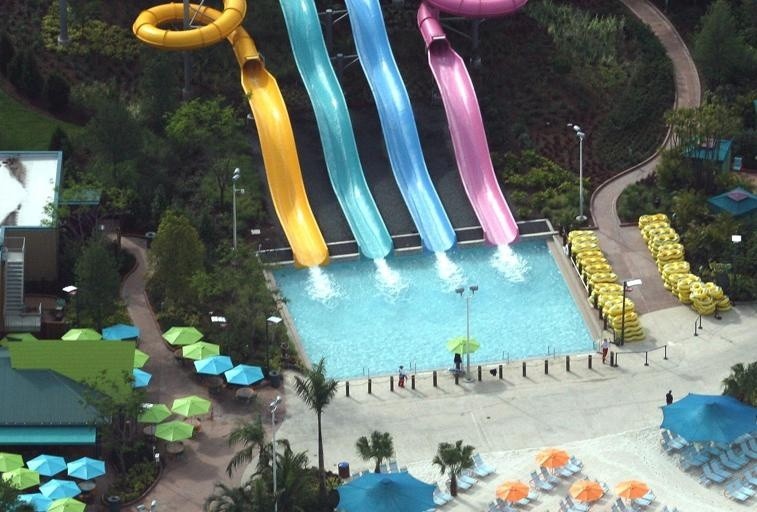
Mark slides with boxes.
[281,0,395,260]
[417,1,526,246]
[347,1,459,253]
[132,0,332,267]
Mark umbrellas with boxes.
[567,477,604,505]
[493,478,531,506]
[656,389,756,445]
[447,334,479,358]
[531,446,569,470]
[613,479,650,501]
[331,470,442,511]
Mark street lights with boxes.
[265,317,282,375]
[730,233,742,307]
[621,279,642,345]
[232,169,240,253]
[269,393,283,511]
[454,285,478,382]
[567,123,585,219]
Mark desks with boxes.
[207,377,223,394]
[143,425,156,443]
[78,481,97,502]
[166,442,184,465]
[236,388,253,405]
[185,418,201,436]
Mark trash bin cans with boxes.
[338,462,350,478]
[108,496,120,512]
[146,232,156,249]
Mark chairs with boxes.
[487,451,585,511]
[348,457,411,481]
[556,475,610,511]
[661,430,756,502]
[430,451,497,511]
[609,482,680,511]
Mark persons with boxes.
[664,390,672,404]
[600,337,610,365]
[183,417,201,440]
[397,365,408,390]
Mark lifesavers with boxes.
[638,213,732,316]
[569,228,646,342]
